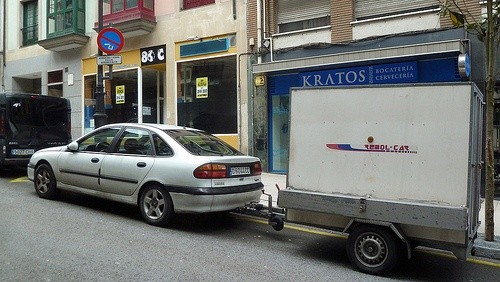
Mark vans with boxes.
[0,92,72,174]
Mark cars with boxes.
[28,122,264,227]
